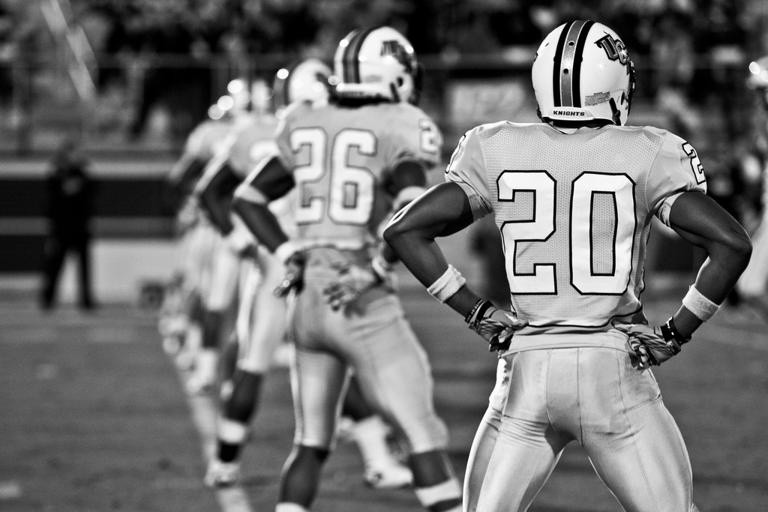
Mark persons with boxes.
[381,21,753,512]
[160,58,415,489]
[89,0,768,146]
[237,22,463,512]
[35,138,94,308]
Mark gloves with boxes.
[323,261,380,311]
[611,318,681,370]
[221,26,415,111]
[272,249,307,298]
[464,298,524,354]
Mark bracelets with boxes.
[682,285,720,323]
[425,264,466,304]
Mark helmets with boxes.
[530,20,642,125]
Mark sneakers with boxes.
[364,463,413,487]
[203,461,240,486]
[160,313,214,393]
